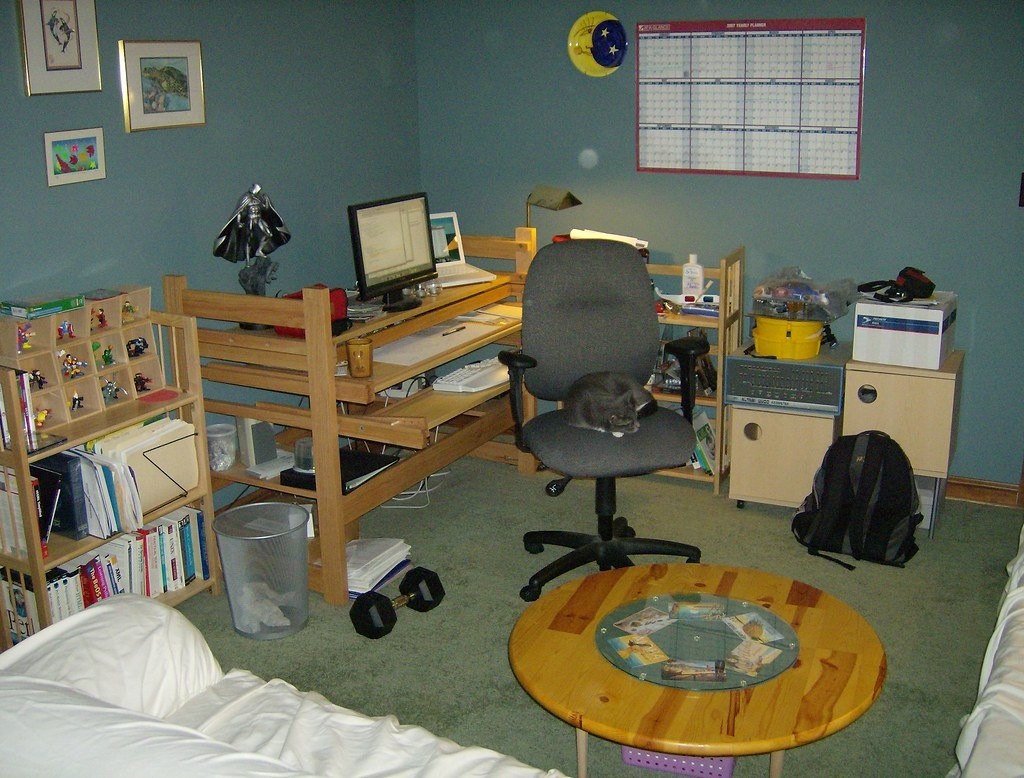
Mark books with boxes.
[2,505,210,645]
[666,404,728,476]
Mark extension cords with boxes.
[380,385,423,398]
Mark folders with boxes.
[279,448,400,495]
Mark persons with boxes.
[16,301,155,428]
[236,184,270,268]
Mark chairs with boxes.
[499,239,702,603]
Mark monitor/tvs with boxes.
[346,192,439,313]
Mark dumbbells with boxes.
[350,565,448,639]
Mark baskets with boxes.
[622,744,735,778]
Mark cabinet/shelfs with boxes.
[170,227,553,603]
[1,311,222,651]
[553,247,746,493]
[0,283,164,441]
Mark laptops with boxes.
[420,211,497,288]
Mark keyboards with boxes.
[433,357,510,393]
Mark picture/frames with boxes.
[18,0,101,97]
[44,128,105,186]
[117,38,206,133]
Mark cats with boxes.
[562,372,657,437]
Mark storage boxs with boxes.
[726,291,962,538]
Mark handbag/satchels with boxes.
[858,267,935,298]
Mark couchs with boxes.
[939,526,1024,778]
[0,586,549,777]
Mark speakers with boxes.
[234,417,278,468]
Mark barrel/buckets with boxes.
[751,316,824,360]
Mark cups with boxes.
[346,336,373,378]
[401,279,443,296]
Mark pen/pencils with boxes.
[443,326,466,337]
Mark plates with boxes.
[566,10,629,79]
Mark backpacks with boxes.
[790,430,924,571]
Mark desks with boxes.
[508,563,889,776]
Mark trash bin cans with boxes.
[212,502,309,640]
